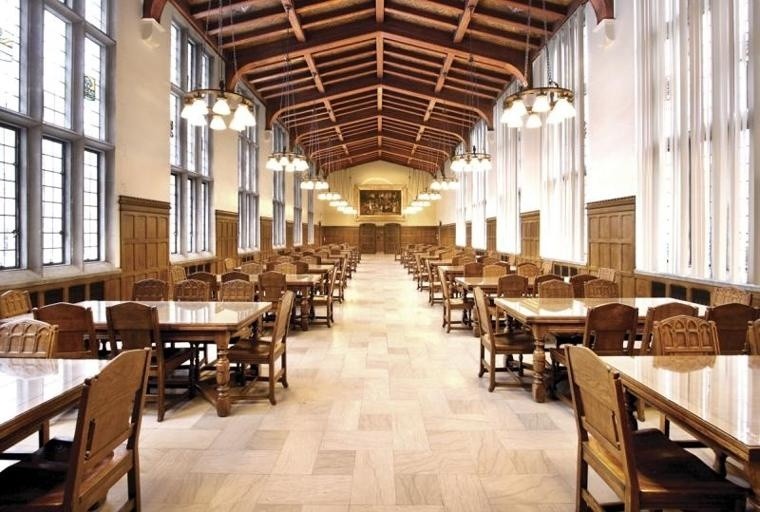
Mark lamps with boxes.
[300,110,329,190]
[180,1,256,132]
[402,209,416,214]
[500,80,577,129]
[407,206,422,211]
[317,191,342,201]
[265,57,309,173]
[430,177,461,190]
[450,145,492,172]
[410,201,431,207]
[344,210,357,214]
[330,200,348,207]
[337,207,353,212]
[418,188,442,200]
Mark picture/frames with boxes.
[353,184,408,222]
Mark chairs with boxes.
[482,257,500,265]
[329,244,334,249]
[226,290,295,406]
[331,250,341,255]
[422,247,429,253]
[515,263,536,275]
[564,345,750,512]
[320,252,329,259]
[456,251,476,258]
[426,260,454,306]
[493,261,510,274]
[307,267,337,328]
[132,279,175,348]
[322,246,329,251]
[241,263,263,274]
[742,319,760,355]
[705,302,760,354]
[162,279,211,381]
[253,252,264,264]
[257,271,296,334]
[429,247,446,256]
[32,302,111,359]
[407,250,418,274]
[291,247,302,258]
[550,302,638,409]
[402,248,412,269]
[0,290,32,319]
[299,256,317,264]
[538,279,594,374]
[290,260,308,274]
[242,261,262,265]
[347,253,352,278]
[355,247,359,264]
[527,274,564,298]
[477,256,489,263]
[597,268,616,283]
[358,248,361,260]
[569,275,600,298]
[488,275,528,334]
[473,286,536,392]
[351,249,356,272]
[276,256,293,263]
[452,255,465,266]
[415,255,434,292]
[274,262,297,275]
[518,264,539,276]
[458,257,477,266]
[406,244,416,250]
[465,263,485,277]
[0,320,59,460]
[222,258,236,273]
[106,302,196,422]
[584,279,638,349]
[336,258,347,288]
[438,267,475,333]
[340,244,345,250]
[625,303,699,356]
[344,242,348,248]
[315,248,322,252]
[653,314,728,479]
[266,261,281,271]
[187,272,218,301]
[0,348,152,511]
[168,266,186,299]
[311,256,321,265]
[439,251,456,260]
[401,247,408,265]
[221,272,259,302]
[303,252,313,257]
[323,259,346,304]
[710,286,753,308]
[195,279,254,381]
[394,246,401,261]
[482,265,506,277]
